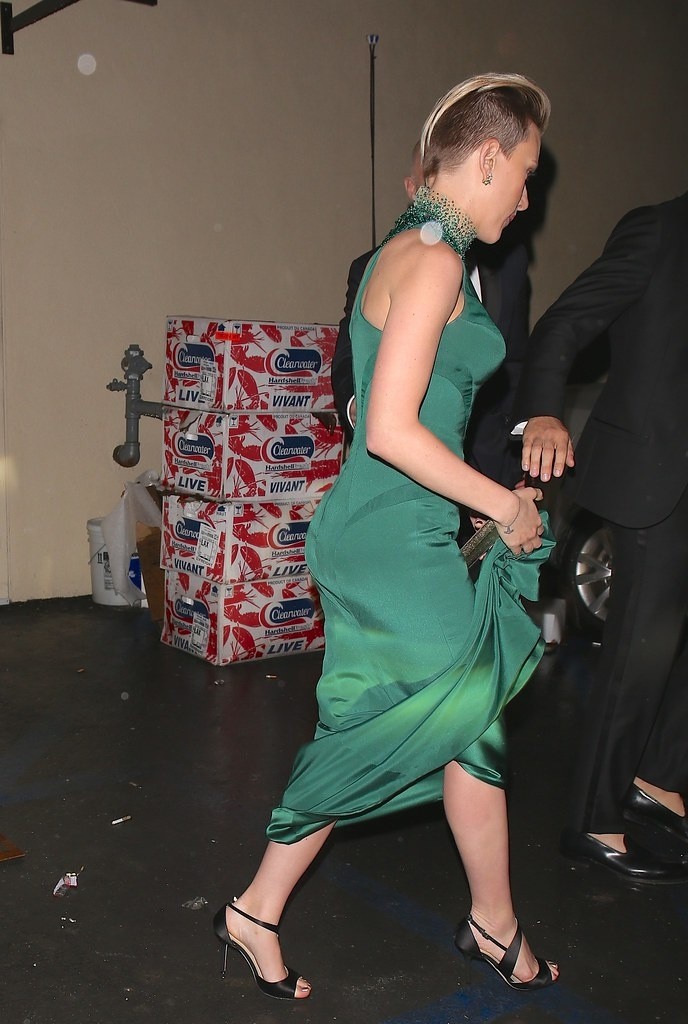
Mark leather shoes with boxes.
[622,782,688,844]
[560,831,688,884]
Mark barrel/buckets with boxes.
[86,516,147,606]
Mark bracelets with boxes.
[500,492,520,535]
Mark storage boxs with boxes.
[161,569,324,666]
[160,405,349,503]
[159,490,335,585]
[161,315,340,414]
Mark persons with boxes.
[211,70,560,1000]
[522,188,688,887]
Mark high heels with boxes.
[453,913,560,991]
[213,900,312,1000]
